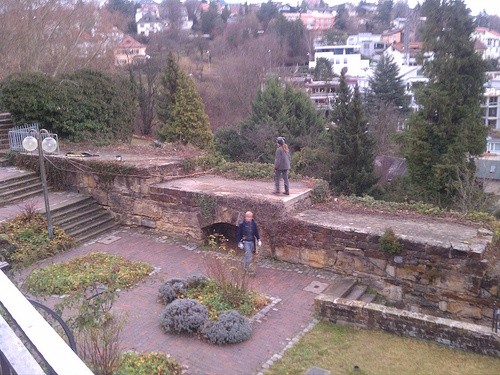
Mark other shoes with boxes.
[282,191,289,195]
[272,191,281,194]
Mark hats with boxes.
[275,136,285,143]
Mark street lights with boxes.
[22,128,58,240]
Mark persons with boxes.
[272,136,291,195]
[237,211,262,273]
